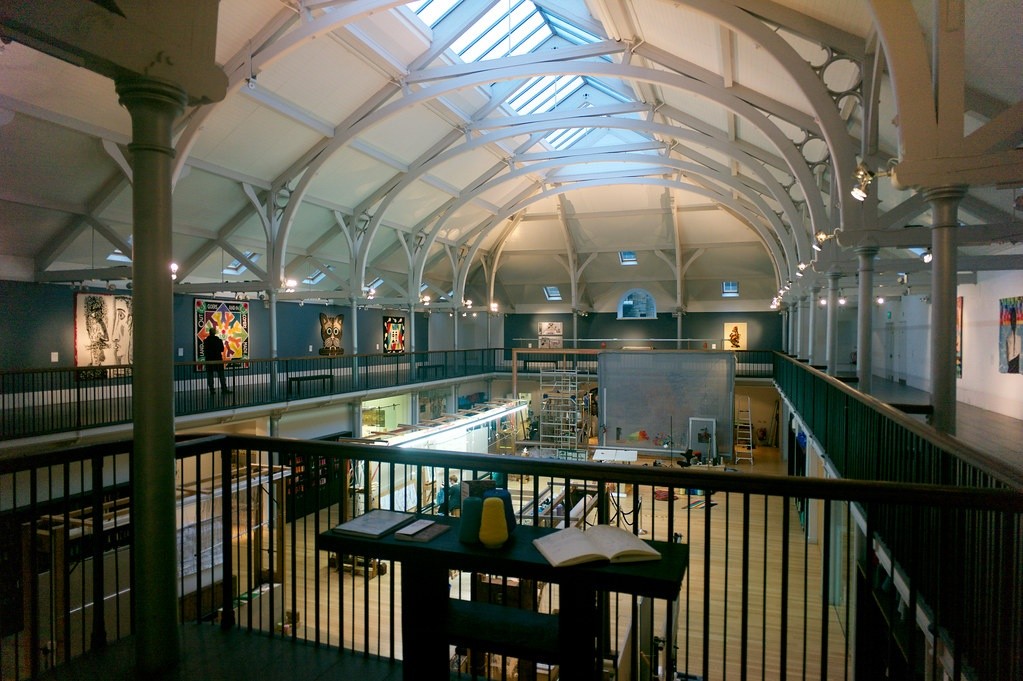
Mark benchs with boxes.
[524,359,558,372]
[289,375,334,398]
[417,364,445,380]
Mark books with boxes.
[330,510,414,539]
[395,519,449,543]
[532,525,662,567]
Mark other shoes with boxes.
[222,390,232,394]
[210,388,215,392]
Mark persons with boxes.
[436,475,460,515]
[204,328,232,394]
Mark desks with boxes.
[318,508,690,681]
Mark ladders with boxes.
[734,396,754,466]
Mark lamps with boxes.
[812,228,840,251]
[795,259,817,277]
[850,162,893,203]
